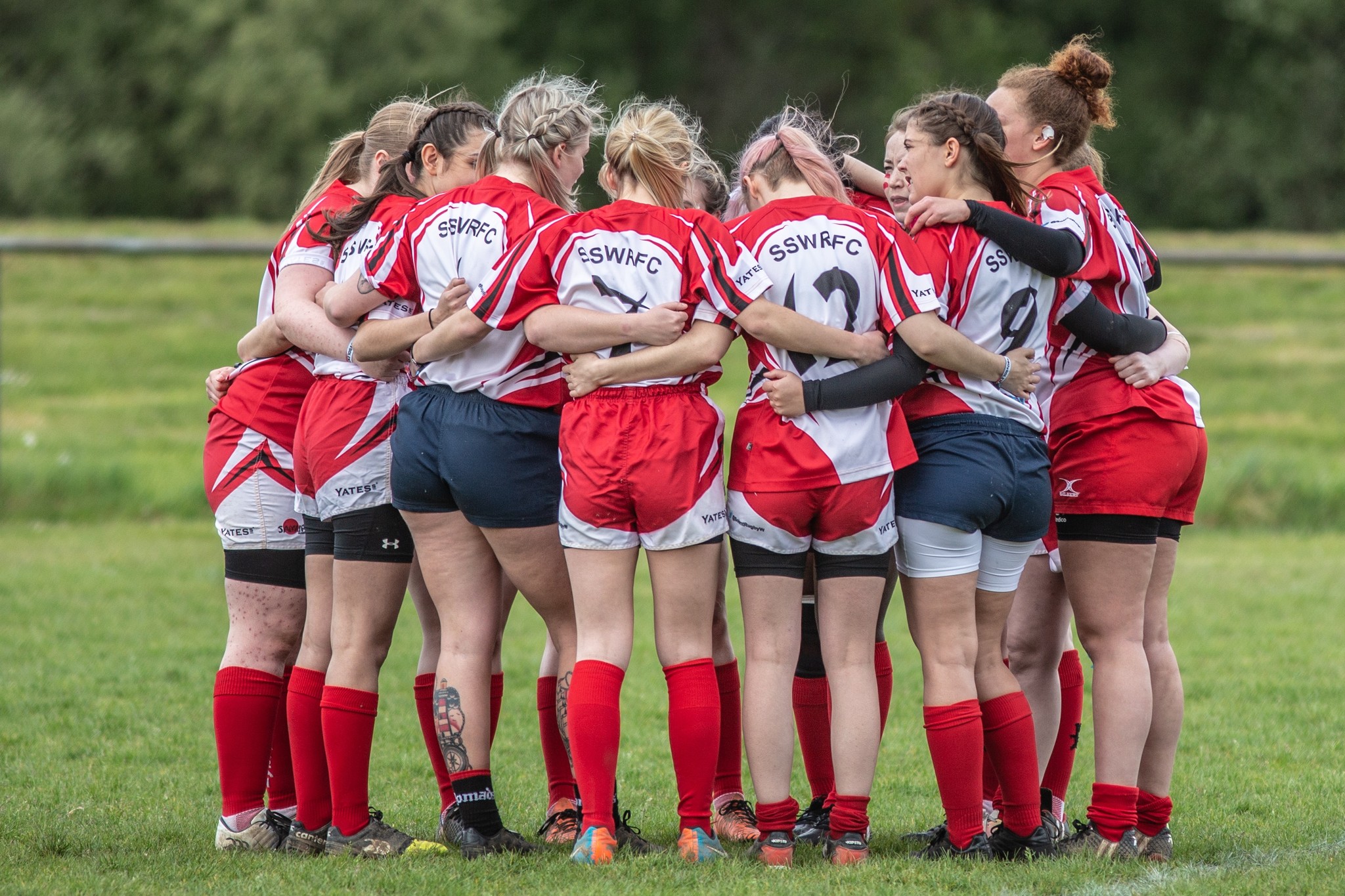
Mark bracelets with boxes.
[233,362,242,368]
[995,356,1011,389]
[346,336,354,363]
[429,308,436,330]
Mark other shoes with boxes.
[900,819,948,845]
[983,808,1002,837]
[1039,786,1071,848]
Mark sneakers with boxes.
[1135,826,1173,860]
[714,794,761,842]
[791,794,834,845]
[436,802,464,852]
[1052,819,1137,862]
[989,824,1051,860]
[914,831,993,862]
[216,807,297,852]
[286,819,331,855]
[326,809,448,859]
[572,810,667,859]
[747,834,796,870]
[545,797,578,844]
[823,826,872,866]
[461,827,539,859]
[570,826,618,866]
[677,827,728,863]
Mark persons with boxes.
[201,35,1210,865]
